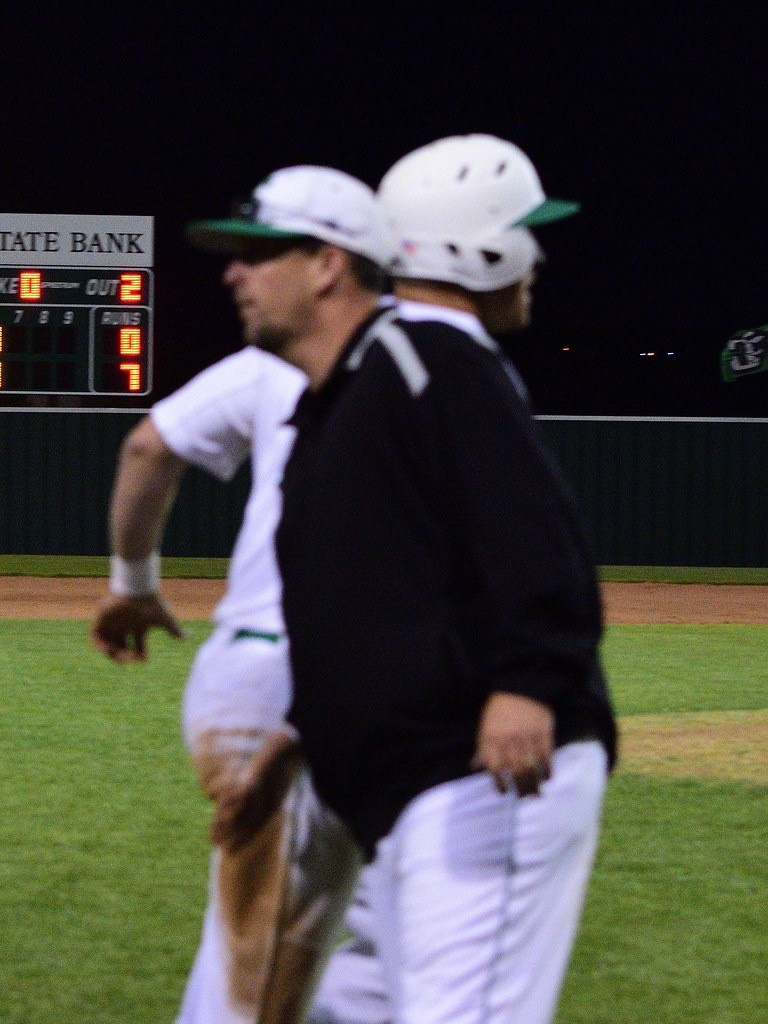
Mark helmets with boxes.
[376,131,581,294]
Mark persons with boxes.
[90,131,577,1024]
[211,165,620,1024]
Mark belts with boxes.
[233,626,285,645]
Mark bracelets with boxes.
[109,550,160,598]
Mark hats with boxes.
[186,165,388,273]
